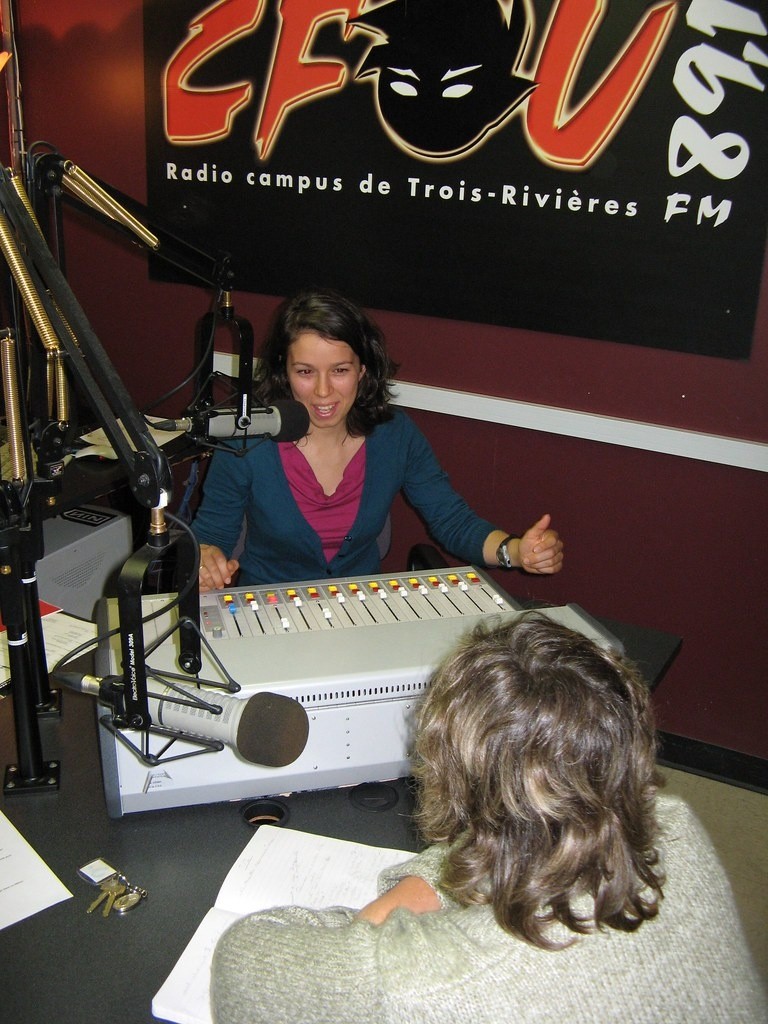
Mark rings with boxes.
[199,565,205,569]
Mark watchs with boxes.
[496,535,520,570]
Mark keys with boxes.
[87,880,125,917]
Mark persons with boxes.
[190,290,565,592]
[210,611,768,1023]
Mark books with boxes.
[151,824,417,1024]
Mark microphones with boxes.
[52,669,309,768]
[154,399,311,443]
